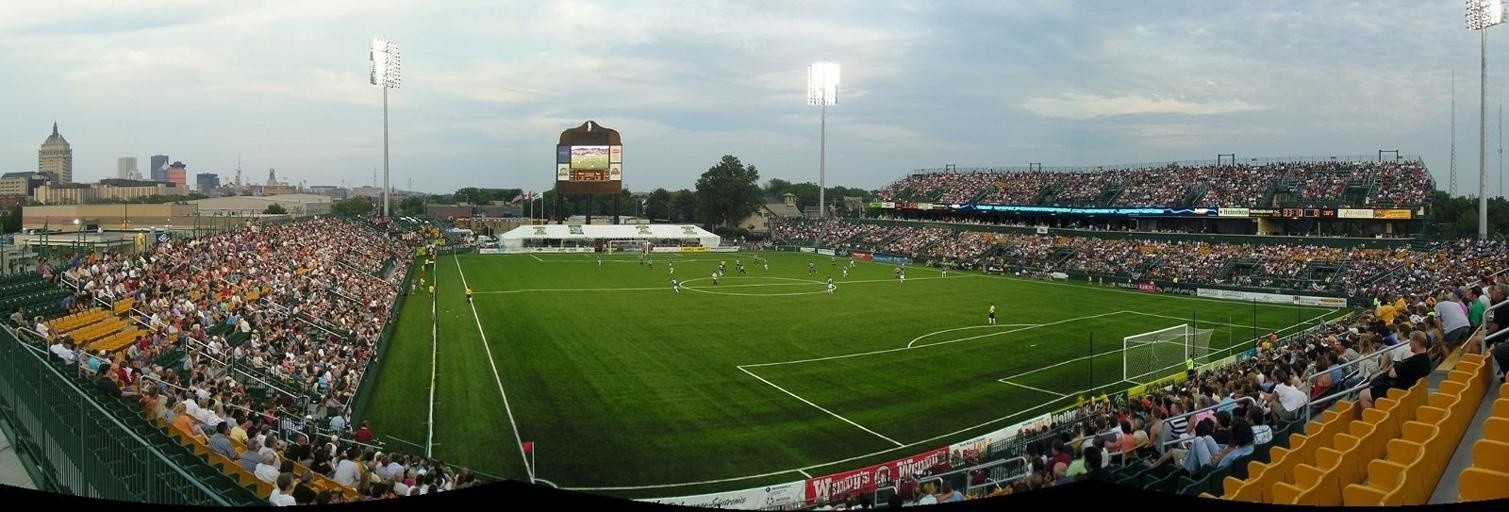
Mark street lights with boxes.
[806,59,842,221]
[72,216,86,251]
[1464,0,1508,246]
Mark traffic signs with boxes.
[372,35,403,218]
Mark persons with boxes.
[731,159,1438,300]
[813,240,1508,511]
[9,209,484,508]
[595,250,727,295]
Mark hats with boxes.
[1347,326,1359,337]
[261,424,272,431]
[330,435,339,442]
[1452,288,1465,298]
[225,375,233,381]
[1323,335,1336,343]
